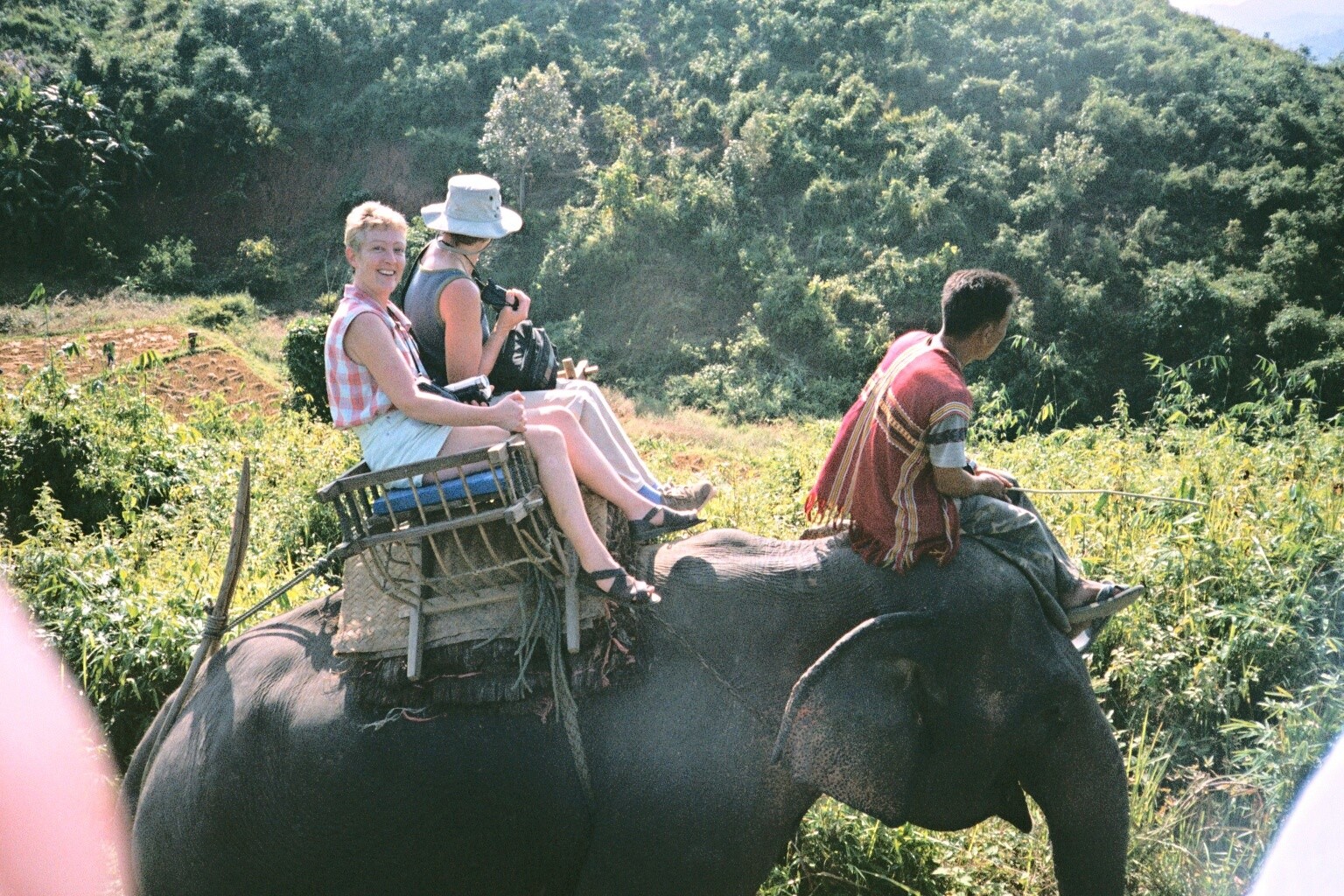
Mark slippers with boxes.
[1067,616,1111,654]
[1065,584,1143,623]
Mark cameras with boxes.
[481,280,520,312]
[443,374,493,403]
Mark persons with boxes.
[404,176,715,513]
[803,270,1144,653]
[325,201,705,607]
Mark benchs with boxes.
[373,375,577,516]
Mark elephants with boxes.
[117,523,1132,896]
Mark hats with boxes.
[421,174,522,239]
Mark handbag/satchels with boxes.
[491,319,557,397]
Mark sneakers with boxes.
[662,481,713,511]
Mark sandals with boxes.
[575,565,662,608]
[627,506,707,543]
